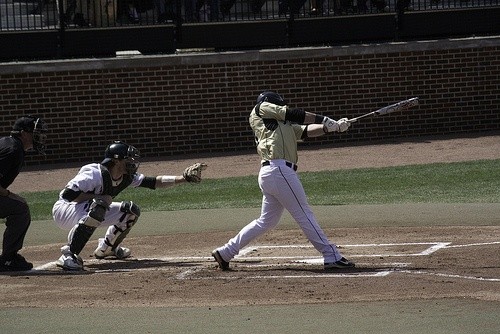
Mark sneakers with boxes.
[0,255,33,271]
[95,245,131,259]
[324,256,355,269]
[56,256,84,270]
[212,249,229,269]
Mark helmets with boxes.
[100,141,132,164]
[256,91,285,106]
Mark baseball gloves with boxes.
[182,162,209,185]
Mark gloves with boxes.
[322,116,338,130]
[336,118,351,133]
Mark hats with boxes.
[11,117,45,133]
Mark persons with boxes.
[52,141,207,271]
[0,117,47,272]
[212,92,355,269]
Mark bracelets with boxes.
[323,126,327,134]
[8,190,10,196]
[315,114,323,124]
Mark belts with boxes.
[263,161,298,171]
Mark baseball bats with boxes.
[322,96,419,135]
